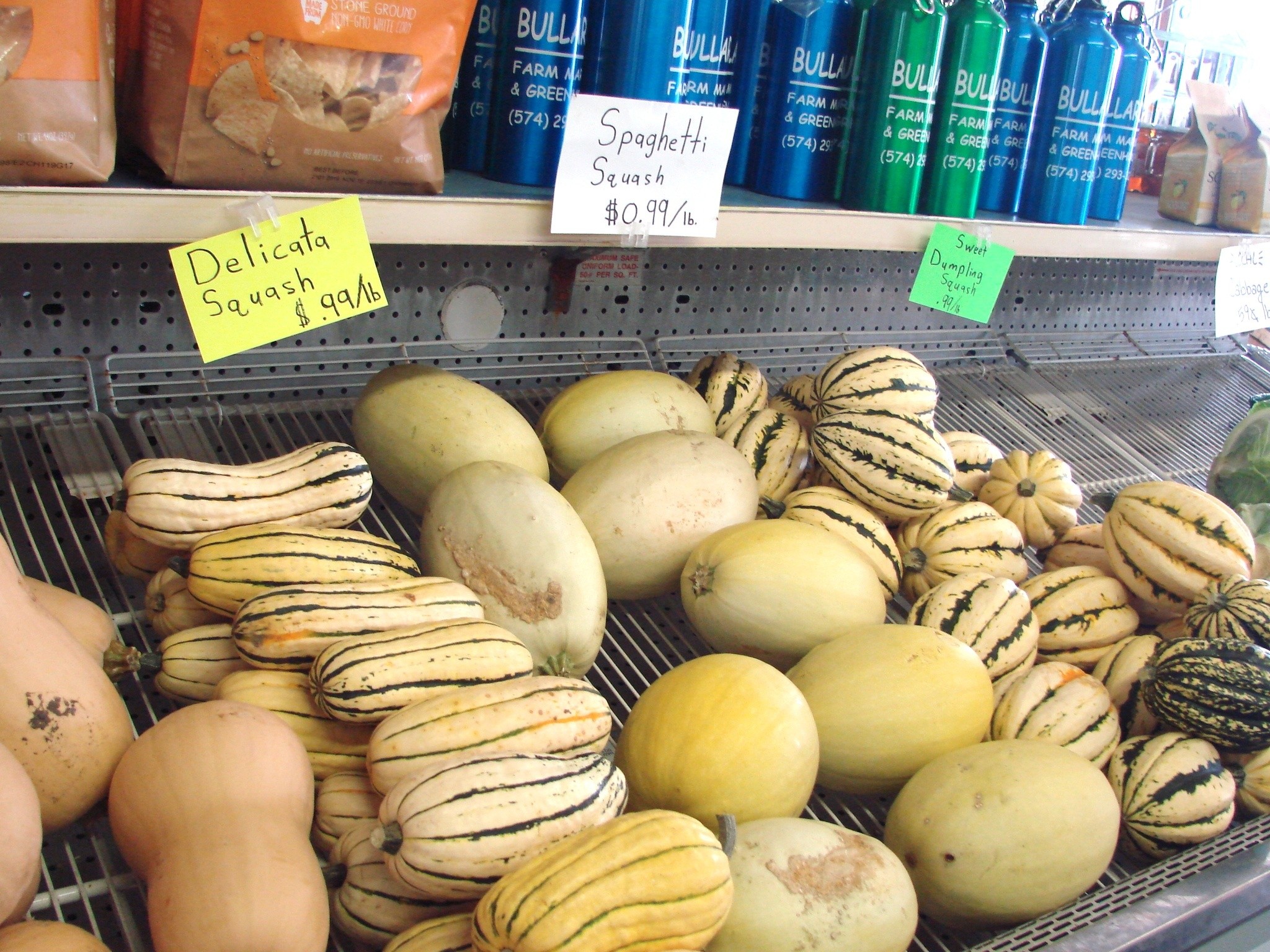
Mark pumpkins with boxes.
[1,348,1270,952]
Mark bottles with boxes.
[1018,0,1118,225]
[1127,121,1191,197]
[1084,1,1151,222]
[441,0,1077,218]
